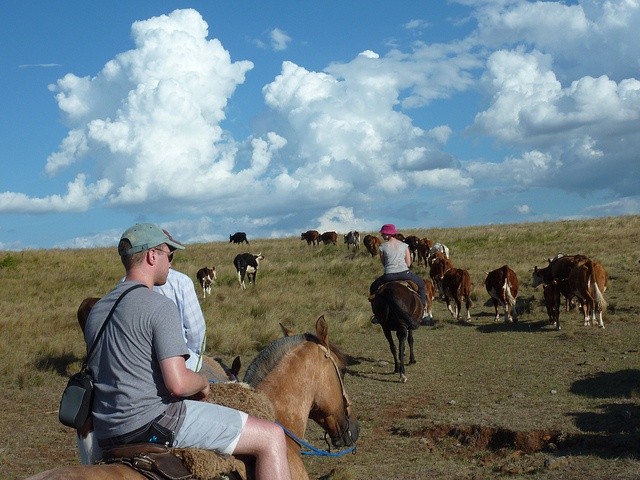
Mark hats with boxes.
[379,224,398,235]
[118,223,186,256]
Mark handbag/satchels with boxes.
[59,285,149,429]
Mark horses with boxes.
[372,282,424,383]
[26,315,360,480]
[213,356,241,383]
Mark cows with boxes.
[417,238,433,269]
[547,253,609,330]
[196,266,217,298]
[301,230,319,246]
[233,252,264,290]
[531,255,608,331]
[343,230,360,252]
[363,235,381,257]
[429,258,453,303]
[405,234,421,262]
[422,279,436,320]
[229,232,249,245]
[318,232,338,246]
[484,264,519,323]
[430,243,449,260]
[428,252,447,269]
[442,268,473,322]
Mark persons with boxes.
[150,228,208,379]
[82,222,294,479]
[368,223,430,324]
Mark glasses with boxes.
[149,247,174,262]
